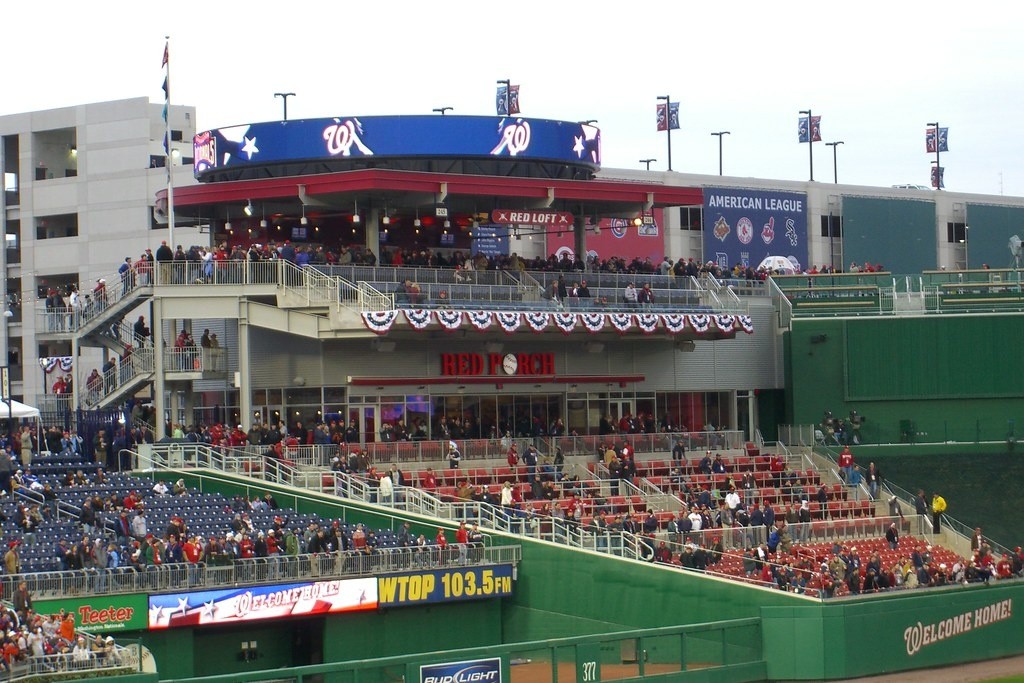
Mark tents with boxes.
[0,395,48,451]
[757,255,795,274]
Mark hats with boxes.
[459,411,1015,584]
[8,631,16,637]
[17,632,22,636]
[65,417,434,551]
[24,631,30,635]
[78,638,84,641]
[56,629,63,634]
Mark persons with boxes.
[0,237,1024,683]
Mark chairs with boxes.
[323,432,972,598]
[0,452,431,574]
